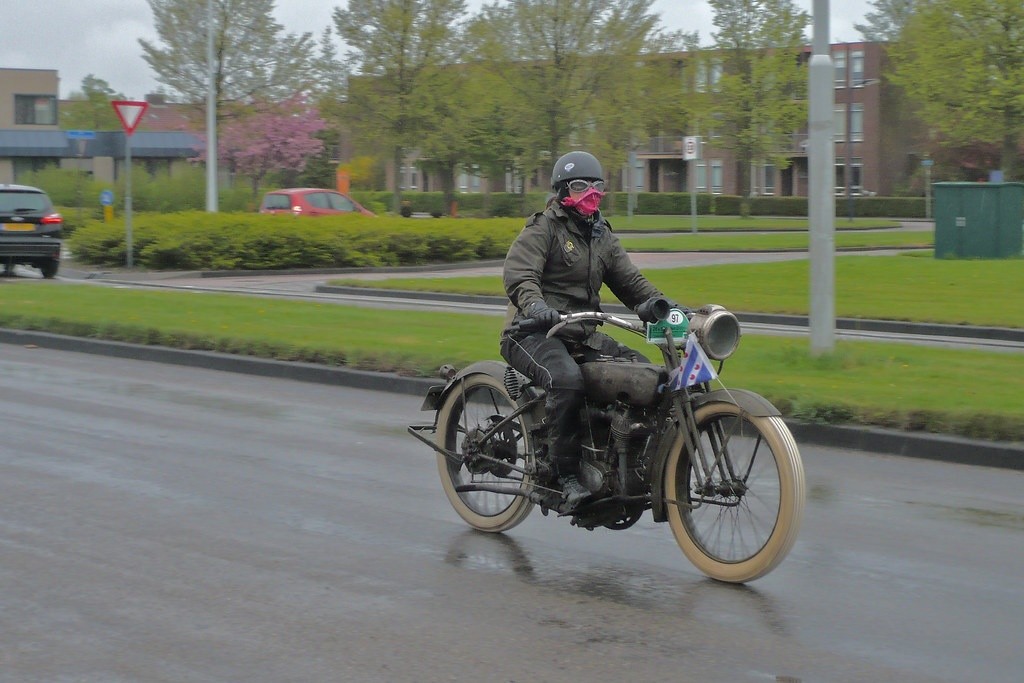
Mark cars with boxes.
[258,187,377,218]
[0,183,63,278]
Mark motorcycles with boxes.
[407,296,805,584]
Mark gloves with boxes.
[529,301,561,331]
[673,303,695,315]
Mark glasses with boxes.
[563,179,604,192]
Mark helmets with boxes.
[551,151,603,189]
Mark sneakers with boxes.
[558,474,593,503]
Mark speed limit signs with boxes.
[683,141,697,156]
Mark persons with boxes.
[499,151,692,505]
[399,198,412,217]
